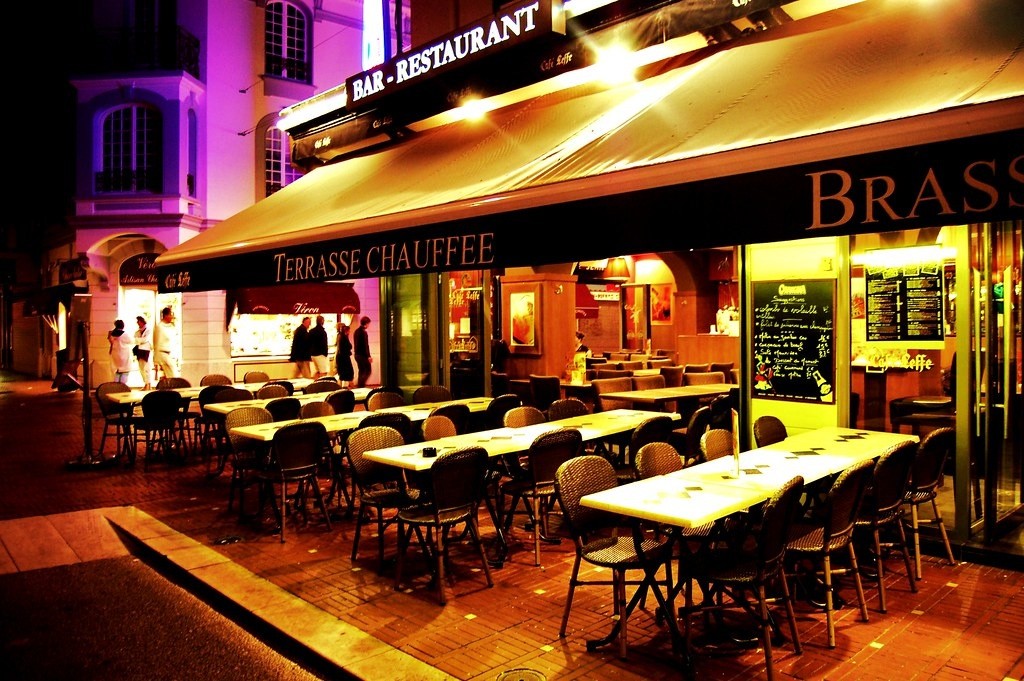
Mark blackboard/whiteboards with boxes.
[750,278,837,407]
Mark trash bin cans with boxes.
[56,349,75,391]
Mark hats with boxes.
[576,331,585,339]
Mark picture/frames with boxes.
[649,282,673,326]
[501,283,542,356]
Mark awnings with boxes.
[153,0,1024,296]
[574,283,600,320]
[23,286,72,335]
[225,282,361,331]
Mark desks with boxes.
[203,387,375,483]
[599,384,741,412]
[229,397,495,531]
[576,426,920,681]
[105,377,315,466]
[361,404,681,588]
[630,368,664,377]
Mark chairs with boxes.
[94,348,987,680]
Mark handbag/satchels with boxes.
[132,344,146,356]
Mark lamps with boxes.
[602,258,631,280]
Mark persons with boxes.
[353,315,372,387]
[334,322,355,388]
[287,317,311,378]
[133,316,152,390]
[308,315,330,379]
[491,329,511,375]
[574,331,589,354]
[152,306,176,379]
[107,319,133,385]
